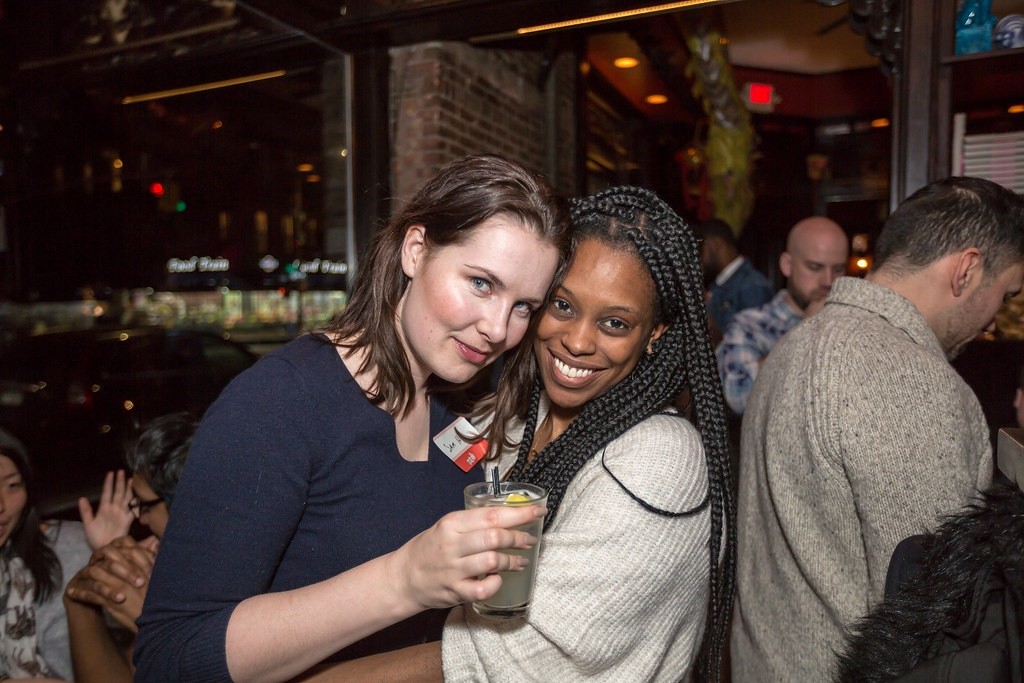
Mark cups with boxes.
[464,481,549,620]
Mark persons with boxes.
[725,174,1023,682]
[0,430,135,683]
[948,289,1023,486]
[697,214,768,339]
[132,154,574,683]
[300,181,735,682]
[714,215,849,413]
[62,409,206,682]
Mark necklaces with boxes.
[531,413,551,449]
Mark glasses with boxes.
[127,497,165,518]
